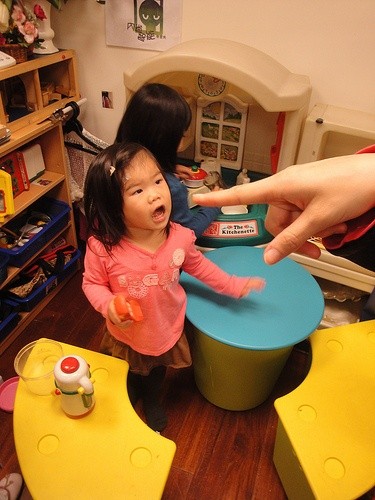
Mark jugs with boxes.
[54,355,96,419]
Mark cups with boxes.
[14,340,63,396]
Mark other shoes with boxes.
[0,473,23,499]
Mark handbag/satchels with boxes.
[7,244,77,299]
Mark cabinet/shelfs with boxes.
[0,48,81,355]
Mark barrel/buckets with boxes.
[200,157,222,177]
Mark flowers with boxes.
[5,6,47,50]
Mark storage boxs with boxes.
[0,198,81,339]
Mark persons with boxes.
[81,143,265,435]
[192,144,375,273]
[115,83,226,238]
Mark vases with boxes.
[0,44,32,63]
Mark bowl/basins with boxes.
[182,165,208,187]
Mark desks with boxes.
[179,246,324,410]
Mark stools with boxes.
[13,336,176,500]
[272,320,375,500]
